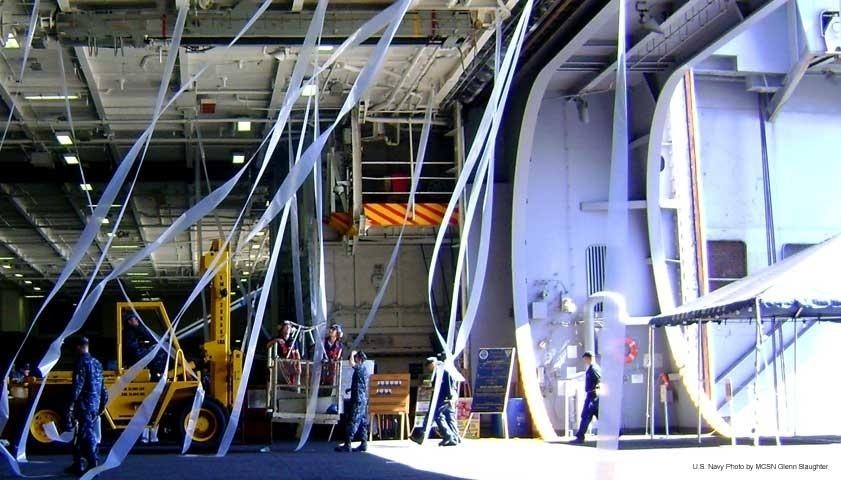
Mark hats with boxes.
[329,324,342,334]
[581,352,593,357]
[423,357,437,364]
[355,350,367,359]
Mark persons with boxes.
[318,324,344,396]
[568,351,624,444]
[266,323,304,392]
[64,336,108,479]
[9,362,31,381]
[334,349,367,453]
[122,313,166,383]
[408,351,461,446]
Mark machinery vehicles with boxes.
[4,236,244,454]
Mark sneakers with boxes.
[352,443,366,452]
[79,459,101,478]
[65,461,84,473]
[334,445,352,453]
[439,437,461,446]
[568,440,585,445]
[408,436,422,445]
